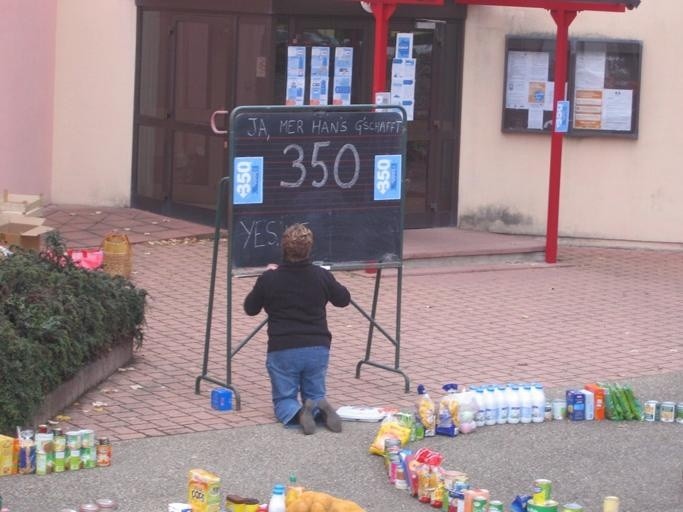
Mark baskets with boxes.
[99,231,133,283]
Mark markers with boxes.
[367,260,382,263]
[321,263,334,266]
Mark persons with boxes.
[243,222,352,434]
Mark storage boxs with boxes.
[0,189,56,252]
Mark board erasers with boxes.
[312,261,324,266]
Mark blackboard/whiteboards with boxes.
[227,105,407,279]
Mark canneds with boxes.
[533,479,552,505]
[472,496,503,512]
[552,399,567,420]
[560,503,584,512]
[643,400,683,423]
[526,499,557,512]
[35,429,112,475]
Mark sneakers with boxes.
[317,395,344,433]
[296,396,316,436]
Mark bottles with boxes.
[96,437,112,468]
[226,473,299,512]
[81,499,116,511]
[470,381,553,426]
